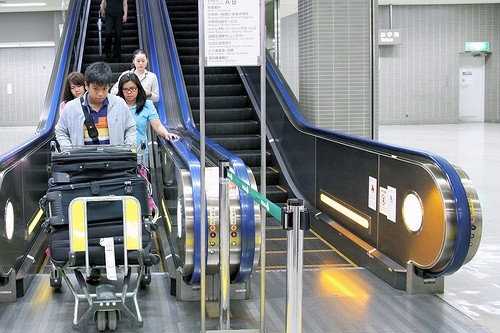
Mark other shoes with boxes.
[103,58,111,62]
[86,268,102,286]
[114,58,125,62]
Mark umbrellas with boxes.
[97,9,103,58]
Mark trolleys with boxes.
[47,140,159,331]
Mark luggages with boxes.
[39,141,162,266]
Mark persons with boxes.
[54,49,181,285]
[100,0,128,62]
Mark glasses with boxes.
[123,88,137,93]
